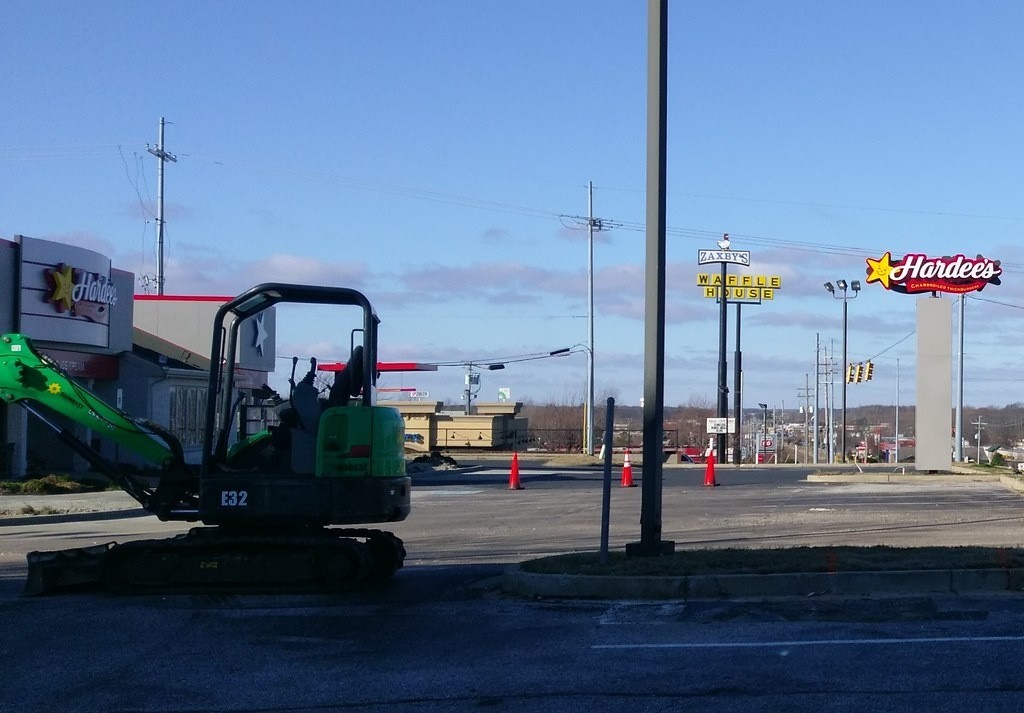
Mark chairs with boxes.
[277,345,365,427]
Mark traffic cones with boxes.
[702,450,721,487]
[504,451,525,490]
[618,449,638,487]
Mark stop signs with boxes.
[758,454,763,463]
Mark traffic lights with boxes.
[847,365,854,382]
[864,362,874,382]
[854,365,864,383]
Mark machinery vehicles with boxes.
[0,282,411,596]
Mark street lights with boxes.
[824,280,861,464]
[759,404,767,455]
